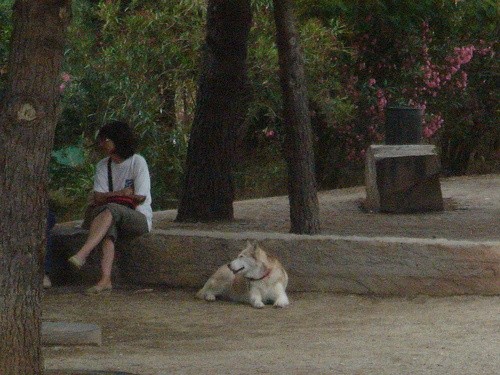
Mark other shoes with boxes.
[44,275,52,288]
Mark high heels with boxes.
[68,255,84,270]
[83,282,112,296]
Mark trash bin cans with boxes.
[382,102,424,145]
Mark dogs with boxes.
[193,238,291,309]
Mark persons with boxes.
[67,120,155,296]
[41,197,58,290]
